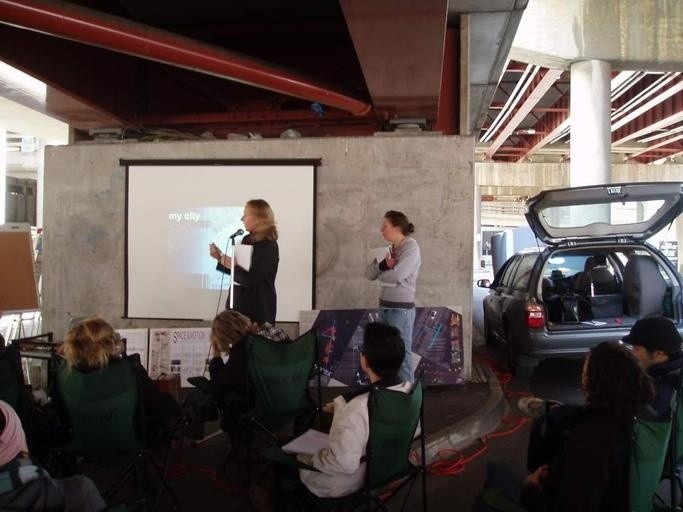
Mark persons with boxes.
[0,400,106,512]
[365,210,422,440]
[34,231,42,286]
[57,315,181,467]
[248,322,422,512]
[208,309,293,491]
[519,341,656,512]
[516,317,683,512]
[211,200,279,329]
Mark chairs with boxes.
[202,329,321,500]
[283,372,426,510]
[52,354,179,510]
[620,413,674,508]
[653,379,682,512]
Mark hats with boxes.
[622,318,683,347]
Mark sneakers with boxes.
[519,396,563,418]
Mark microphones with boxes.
[229,229,244,239]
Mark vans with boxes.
[477,182,683,380]
